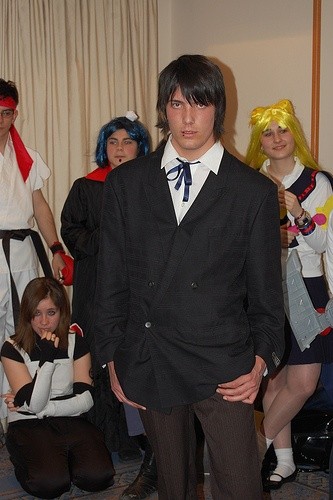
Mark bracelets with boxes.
[294,208,305,220]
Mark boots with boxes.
[124,449,160,500]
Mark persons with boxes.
[88,53,287,500]
[0,276,116,500]
[244,99,333,490]
[60,116,161,463]
[0,71,75,432]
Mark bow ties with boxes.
[166,159,203,202]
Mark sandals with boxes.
[267,467,299,487]
